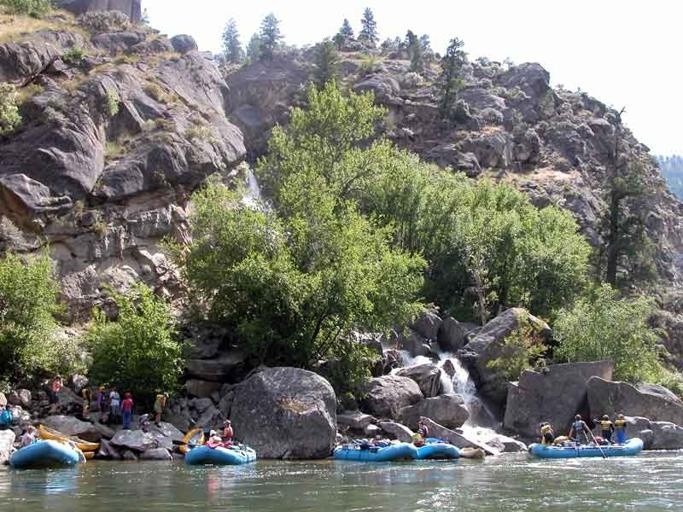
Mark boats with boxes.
[410,439,458,461]
[530,438,644,460]
[182,444,256,466]
[179,428,205,454]
[37,426,99,459]
[334,441,411,462]
[9,440,80,470]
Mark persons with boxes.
[614,413,628,443]
[537,412,554,445]
[568,412,593,445]
[359,419,432,451]
[0,375,245,450]
[592,414,615,440]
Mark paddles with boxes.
[171,439,201,445]
[590,433,608,458]
